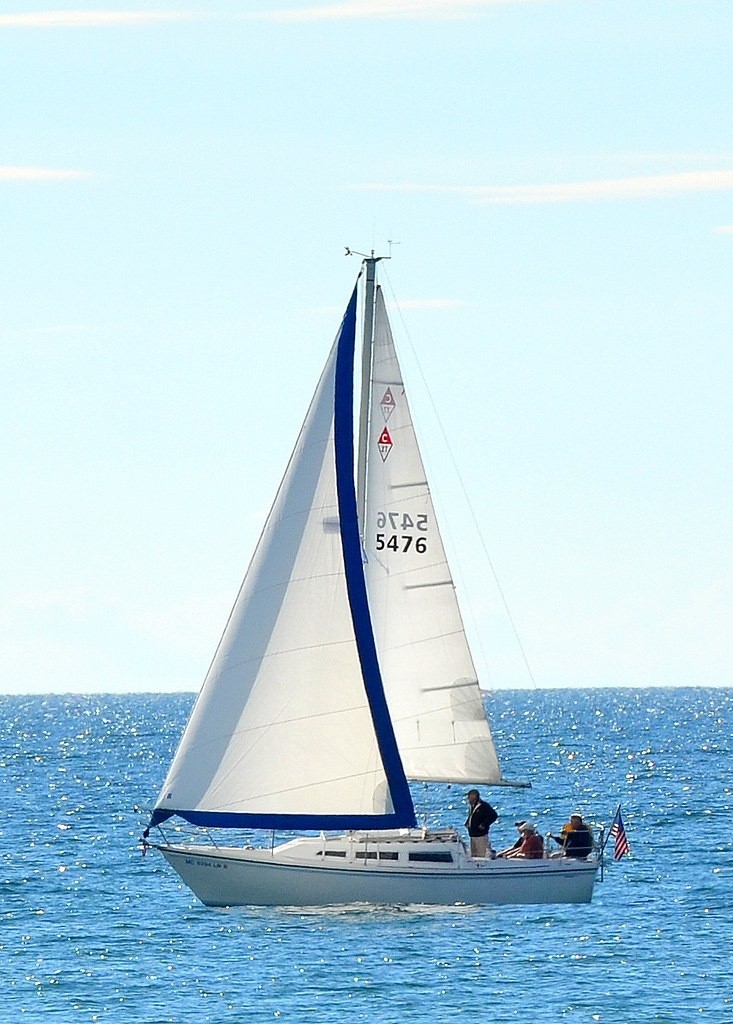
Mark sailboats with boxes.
[139,238,628,905]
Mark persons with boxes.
[462,789,498,857]
[497,821,544,859]
[546,814,592,858]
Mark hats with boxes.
[463,789,479,795]
[570,813,583,823]
[518,823,534,832]
[515,820,528,826]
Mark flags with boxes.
[610,811,628,861]
